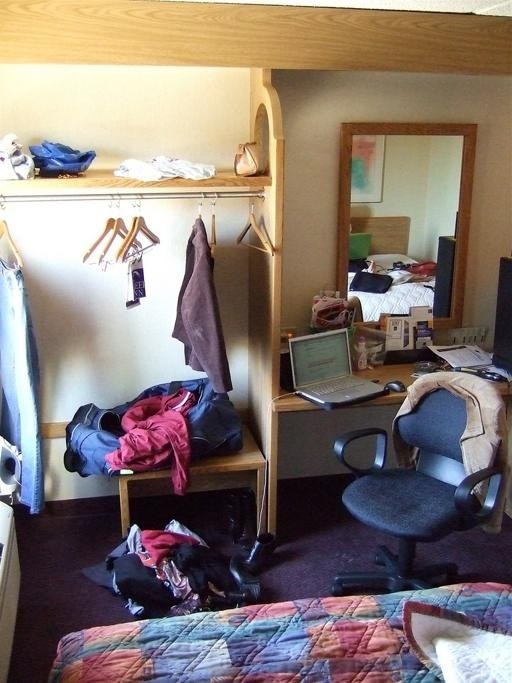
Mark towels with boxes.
[111,151,217,180]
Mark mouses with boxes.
[386,381,405,392]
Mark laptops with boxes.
[288,328,390,410]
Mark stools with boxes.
[111,423,267,542]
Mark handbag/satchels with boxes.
[63,377,243,477]
[233,140,267,176]
[408,260,436,274]
[349,270,393,293]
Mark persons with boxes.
[348,257,376,274]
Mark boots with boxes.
[247,532,274,564]
[214,488,256,543]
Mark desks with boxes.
[274,349,511,534]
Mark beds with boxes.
[50,574,509,681]
[347,215,437,321]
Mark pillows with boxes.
[365,251,418,271]
[401,599,511,683]
[387,268,413,284]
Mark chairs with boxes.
[326,370,511,595]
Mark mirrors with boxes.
[336,121,479,332]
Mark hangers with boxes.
[234,190,275,256]
[204,192,223,260]
[1,196,25,268]
[195,192,205,224]
[79,195,162,264]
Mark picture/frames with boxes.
[351,132,387,204]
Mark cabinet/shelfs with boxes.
[1,64,286,551]
